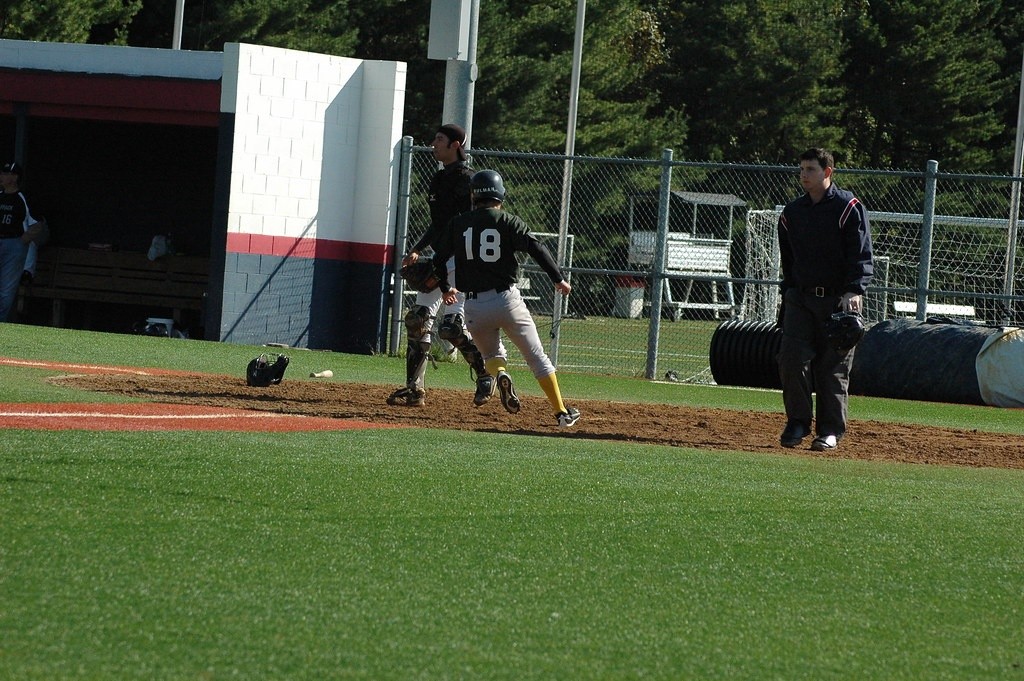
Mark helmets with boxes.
[472,170,505,203]
[247,353,289,387]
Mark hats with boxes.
[0,161,21,173]
[437,123,467,162]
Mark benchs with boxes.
[892,301,986,324]
[16,244,210,337]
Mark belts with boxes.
[803,285,835,297]
[467,286,510,300]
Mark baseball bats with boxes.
[310,370,334,378]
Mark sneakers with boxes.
[473,374,497,407]
[386,388,425,406]
[781,423,812,447]
[497,370,520,414]
[812,433,845,451]
[556,407,580,427]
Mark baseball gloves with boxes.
[398,259,441,294]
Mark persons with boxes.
[385,124,497,407]
[0,162,42,322]
[432,169,580,428]
[777,148,874,449]
